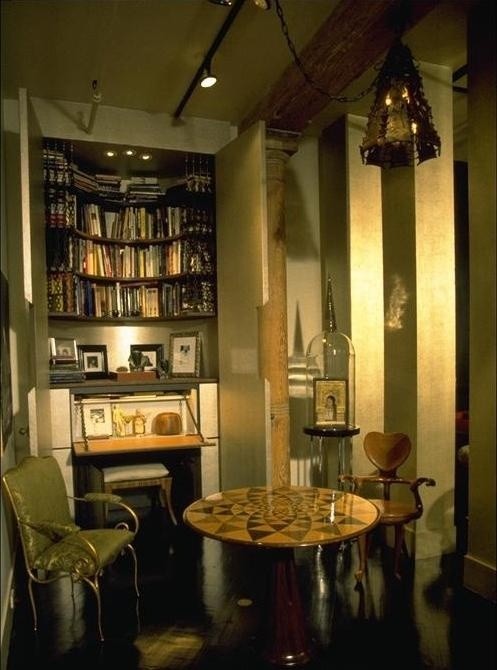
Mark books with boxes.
[69,164,189,319]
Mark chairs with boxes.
[336,432,435,585]
[2,454,141,644]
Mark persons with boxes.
[324,396,338,421]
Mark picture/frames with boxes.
[51,337,78,365]
[78,344,108,378]
[131,344,164,377]
[169,332,202,377]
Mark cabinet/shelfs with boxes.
[41,140,215,323]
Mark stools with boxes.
[101,460,179,528]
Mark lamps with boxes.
[199,62,216,88]
[272,0,440,171]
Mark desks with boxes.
[183,486,380,668]
